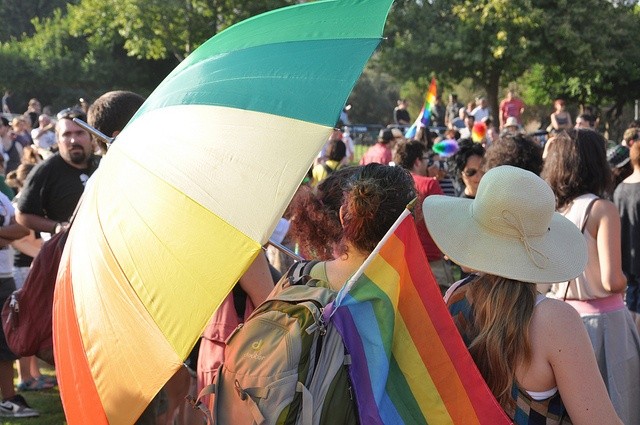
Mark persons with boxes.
[78,96,92,112]
[623,126,639,148]
[342,127,354,167]
[499,116,526,137]
[86,90,145,155]
[536,129,640,424]
[457,115,475,138]
[444,93,464,126]
[574,114,599,132]
[318,128,339,162]
[470,97,491,122]
[262,163,417,425]
[292,165,363,262]
[6,163,59,392]
[31,113,59,155]
[499,87,528,131]
[420,165,624,425]
[430,93,447,135]
[392,98,412,136]
[312,139,346,187]
[612,141,640,336]
[414,126,436,167]
[455,140,488,280]
[394,140,456,296]
[15,107,103,235]
[186,248,284,425]
[629,120,640,128]
[9,117,33,158]
[0,117,24,174]
[467,101,476,113]
[391,128,406,148]
[488,127,500,144]
[607,144,634,200]
[338,126,347,169]
[550,98,572,135]
[1,89,14,122]
[340,104,351,125]
[43,105,52,116]
[446,129,461,140]
[474,94,484,104]
[481,134,546,177]
[482,116,496,140]
[20,145,54,166]
[428,130,438,144]
[359,128,393,166]
[0,151,16,202]
[433,124,447,144]
[23,97,40,129]
[0,192,42,419]
[452,106,467,128]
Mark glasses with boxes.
[462,166,487,177]
[419,156,434,166]
[57,107,83,117]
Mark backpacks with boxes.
[3,214,69,367]
[185,260,359,424]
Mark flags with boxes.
[320,208,513,425]
[404,75,437,139]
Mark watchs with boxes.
[53,222,62,234]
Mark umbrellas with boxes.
[52,0,394,425]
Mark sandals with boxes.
[19,377,54,391]
[41,374,57,384]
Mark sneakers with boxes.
[0,394,39,417]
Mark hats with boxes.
[375,129,395,138]
[504,116,519,127]
[422,164,588,283]
[390,127,400,135]
[607,144,631,169]
